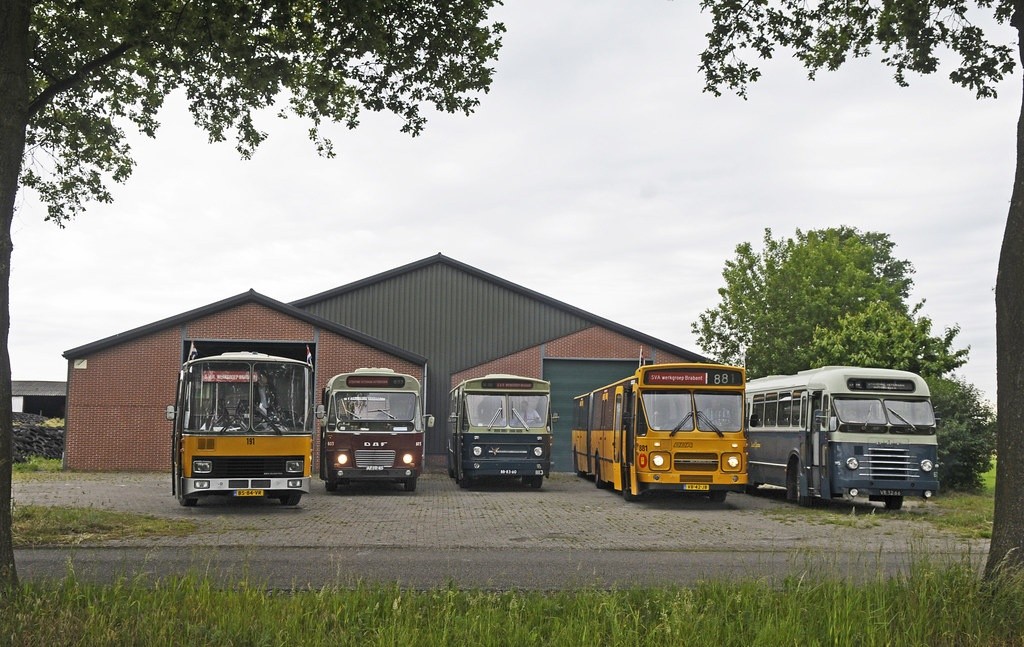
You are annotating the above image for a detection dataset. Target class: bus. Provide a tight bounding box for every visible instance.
[165,350,327,507]
[445,374,561,491]
[317,367,436,492]
[571,363,762,503]
[735,365,943,511]
